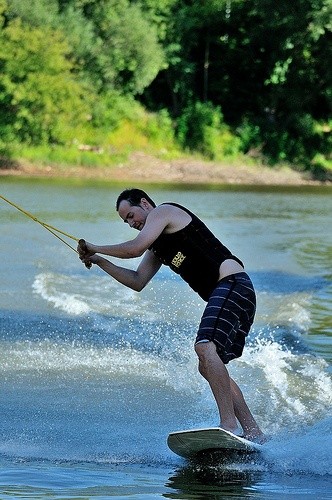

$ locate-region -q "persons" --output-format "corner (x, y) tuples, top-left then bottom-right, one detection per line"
(76, 188), (271, 449)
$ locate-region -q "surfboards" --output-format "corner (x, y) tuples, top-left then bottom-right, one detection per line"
(168, 427), (262, 459)
